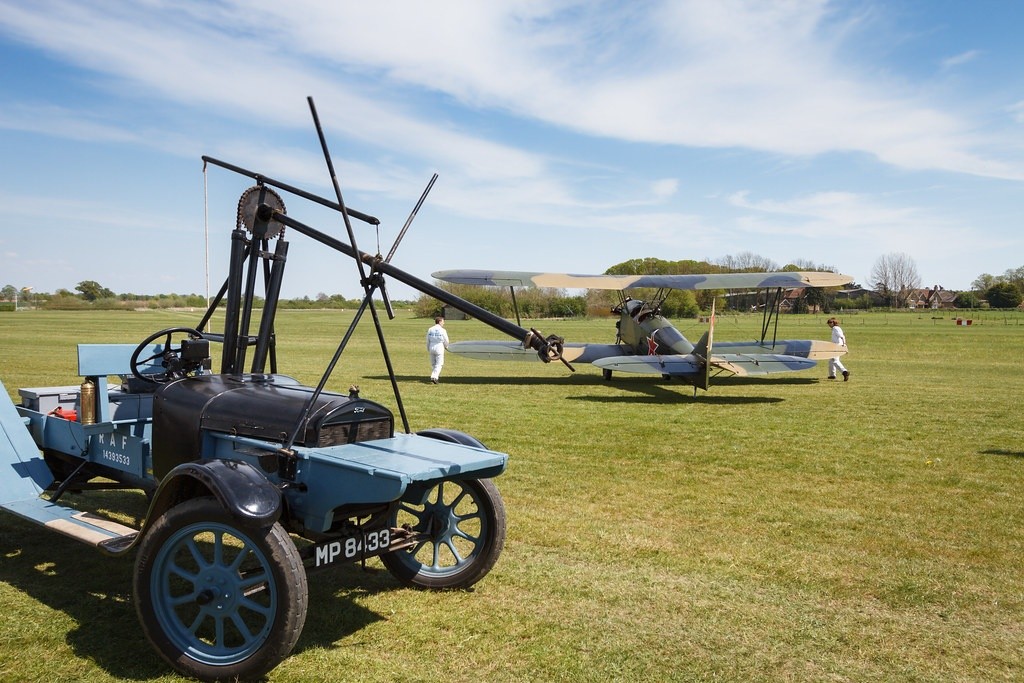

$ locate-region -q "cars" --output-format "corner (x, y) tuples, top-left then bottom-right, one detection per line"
(0, 95), (578, 682)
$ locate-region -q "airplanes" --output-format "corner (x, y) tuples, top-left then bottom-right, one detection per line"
(429, 270), (853, 392)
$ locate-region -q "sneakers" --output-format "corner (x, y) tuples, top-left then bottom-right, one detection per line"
(842, 370), (850, 380)
(430, 378), (440, 384)
(828, 376), (836, 379)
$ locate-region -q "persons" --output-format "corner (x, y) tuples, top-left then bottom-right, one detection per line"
(426, 317), (450, 385)
(826, 317), (850, 382)
